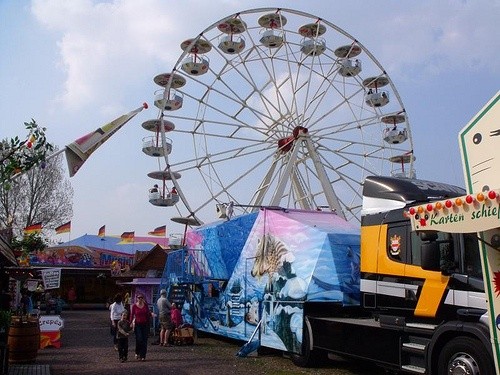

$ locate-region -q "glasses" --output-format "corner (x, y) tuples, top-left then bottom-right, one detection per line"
(137, 297), (144, 299)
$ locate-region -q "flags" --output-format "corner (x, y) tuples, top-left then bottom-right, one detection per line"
(148, 225), (166, 236)
(55, 221), (70, 234)
(98, 225), (105, 237)
(121, 232), (134, 243)
(23, 222), (42, 234)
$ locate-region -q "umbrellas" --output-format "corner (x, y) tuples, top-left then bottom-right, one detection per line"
(171, 217), (202, 246)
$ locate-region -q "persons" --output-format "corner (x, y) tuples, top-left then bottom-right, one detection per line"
(153, 303), (181, 345)
(367, 89), (373, 95)
(157, 289), (174, 347)
(355, 59), (360, 67)
(129, 294), (157, 361)
(171, 187), (177, 194)
(116, 310), (133, 363)
(0, 287), (77, 316)
(106, 292), (131, 351)
(151, 184), (158, 193)
(382, 92), (387, 98)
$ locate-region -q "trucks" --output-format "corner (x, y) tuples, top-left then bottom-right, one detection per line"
(286, 176), (496, 375)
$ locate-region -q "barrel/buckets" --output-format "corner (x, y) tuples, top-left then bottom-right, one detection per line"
(6, 315), (40, 361)
